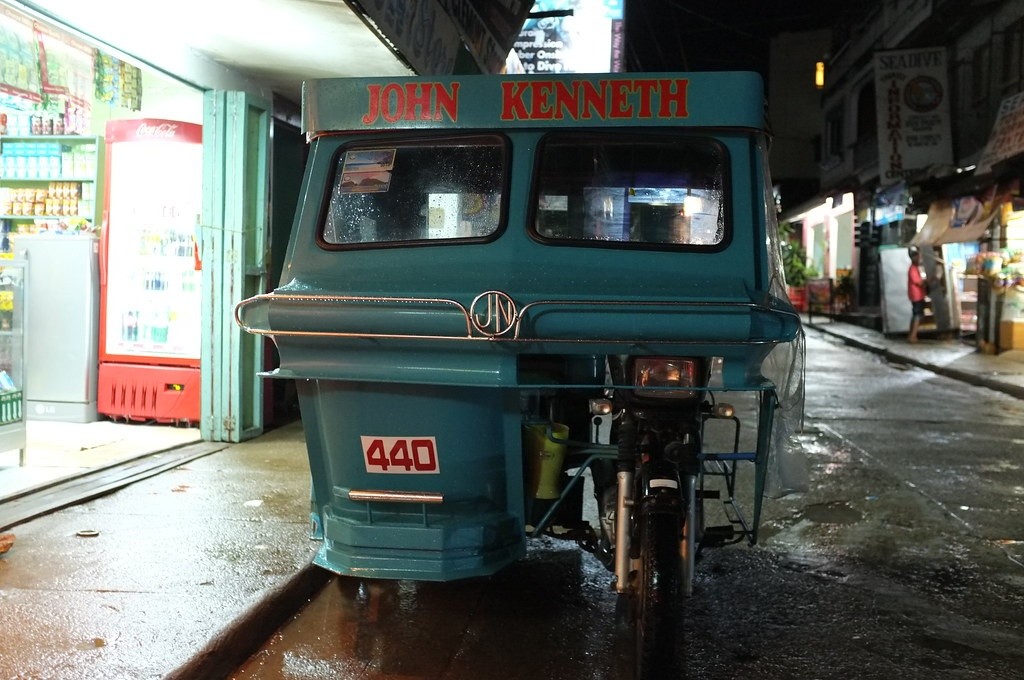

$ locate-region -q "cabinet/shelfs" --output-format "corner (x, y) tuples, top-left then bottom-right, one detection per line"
(0, 5), (141, 468)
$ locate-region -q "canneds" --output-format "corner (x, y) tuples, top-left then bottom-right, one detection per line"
(4, 181), (80, 217)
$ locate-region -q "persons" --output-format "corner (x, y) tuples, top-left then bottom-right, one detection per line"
(908, 246), (929, 344)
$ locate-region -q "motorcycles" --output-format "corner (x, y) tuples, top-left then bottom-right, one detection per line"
(232, 72), (807, 679)
